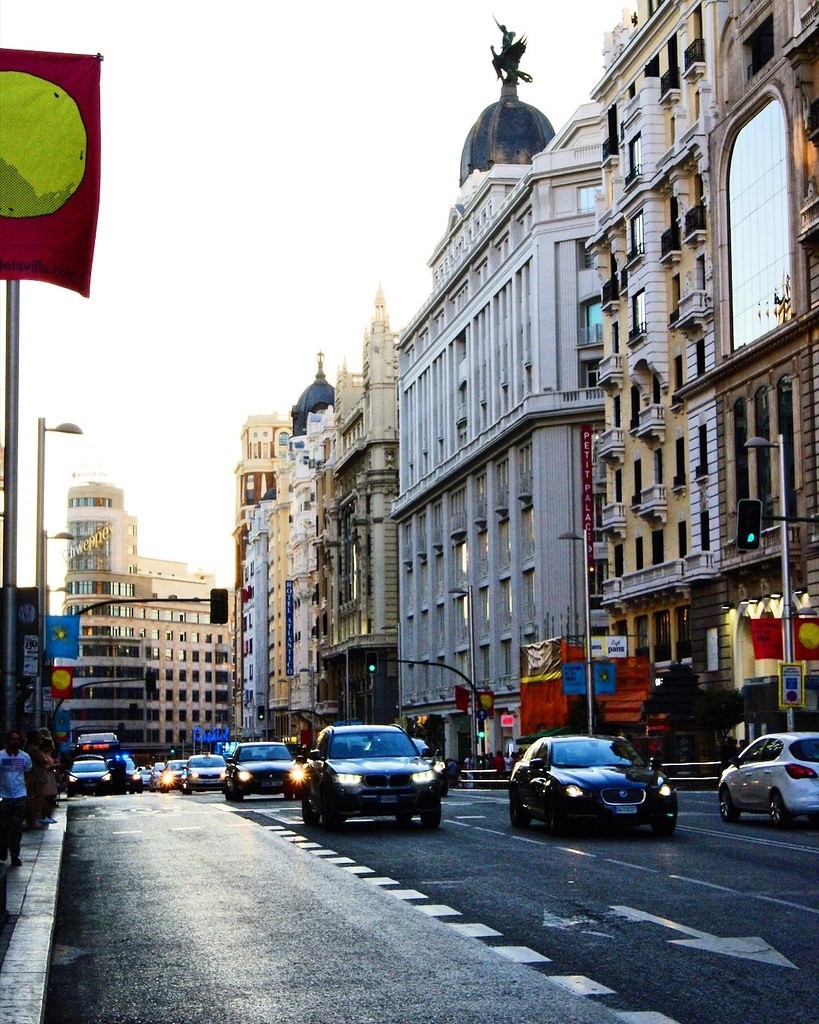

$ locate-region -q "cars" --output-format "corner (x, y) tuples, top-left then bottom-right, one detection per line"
(716, 731), (819, 828)
(123, 758), (143, 794)
(301, 724), (442, 829)
(506, 734), (679, 837)
(64, 759), (115, 797)
(140, 754), (227, 795)
(410, 738), (450, 796)
(223, 741), (303, 803)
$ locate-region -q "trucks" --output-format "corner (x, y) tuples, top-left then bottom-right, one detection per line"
(76, 732), (127, 795)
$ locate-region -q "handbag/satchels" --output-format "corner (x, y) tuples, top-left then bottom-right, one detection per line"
(40, 773), (58, 796)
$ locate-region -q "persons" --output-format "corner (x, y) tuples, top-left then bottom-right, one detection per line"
(723, 737), (746, 764)
(446, 748), (527, 789)
(20, 728), (63, 828)
(495, 20), (512, 52)
(0, 731), (31, 866)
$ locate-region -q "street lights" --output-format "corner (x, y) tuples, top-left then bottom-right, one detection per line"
(449, 585), (478, 789)
(36, 416), (84, 743)
(45, 583), (71, 617)
(743, 433), (795, 734)
(256, 665), (317, 749)
(557, 529), (594, 735)
(37, 530), (75, 737)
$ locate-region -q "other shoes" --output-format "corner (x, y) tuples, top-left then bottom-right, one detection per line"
(11, 855), (22, 866)
(27, 821), (44, 830)
(0, 842), (8, 862)
(39, 816), (57, 823)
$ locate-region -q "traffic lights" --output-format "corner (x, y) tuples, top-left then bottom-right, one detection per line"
(736, 499), (763, 551)
(170, 745), (176, 754)
(364, 651), (378, 674)
(333, 649), (350, 725)
(258, 706), (265, 721)
